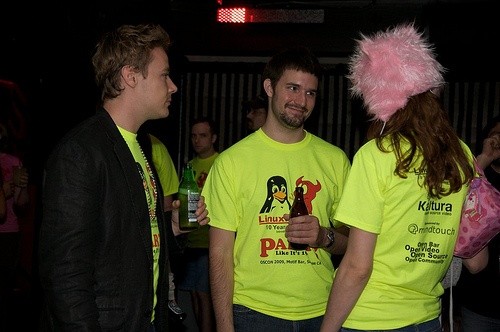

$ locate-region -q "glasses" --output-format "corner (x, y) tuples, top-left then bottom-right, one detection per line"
(246, 109), (268, 116)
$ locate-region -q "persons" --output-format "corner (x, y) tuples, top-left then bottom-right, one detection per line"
(180, 113), (220, 331)
(146, 130), (180, 212)
(200, 46), (352, 331)
(243, 91), (270, 132)
(0, 150), (35, 275)
(473, 124), (499, 186)
(42, 18), (211, 331)
(318, 21), (499, 331)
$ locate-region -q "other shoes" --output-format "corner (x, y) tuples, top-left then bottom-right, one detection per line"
(167, 301), (184, 314)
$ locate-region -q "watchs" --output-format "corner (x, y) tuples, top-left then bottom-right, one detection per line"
(324, 226), (337, 249)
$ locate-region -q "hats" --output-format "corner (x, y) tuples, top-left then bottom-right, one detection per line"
(241, 94), (268, 108)
(345, 21), (447, 123)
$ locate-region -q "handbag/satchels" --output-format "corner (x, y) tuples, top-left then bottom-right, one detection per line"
(453, 159), (500, 259)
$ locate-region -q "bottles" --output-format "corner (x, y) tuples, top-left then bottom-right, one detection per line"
(179, 162), (200, 231)
(289, 187), (310, 251)
